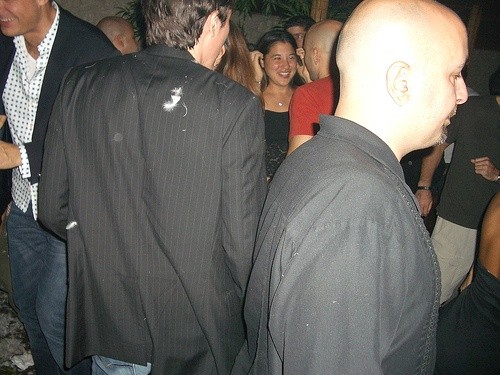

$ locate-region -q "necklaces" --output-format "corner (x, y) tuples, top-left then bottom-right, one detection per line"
(278, 101), (283, 106)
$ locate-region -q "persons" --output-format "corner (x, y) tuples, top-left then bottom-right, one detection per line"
(249, 30), (312, 182)
(39, 0), (267, 375)
(0, 0), (123, 375)
(434, 192), (500, 375)
(402, 145), (444, 234)
(284, 16), (317, 50)
(285, 20), (344, 156)
(211, 19), (265, 114)
(231, 0), (468, 375)
(415, 71), (500, 305)
(97, 16), (139, 55)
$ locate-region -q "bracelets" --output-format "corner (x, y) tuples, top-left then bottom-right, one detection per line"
(254, 80), (262, 84)
(495, 171), (500, 183)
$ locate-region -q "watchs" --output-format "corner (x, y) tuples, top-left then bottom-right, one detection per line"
(417, 186), (432, 190)
